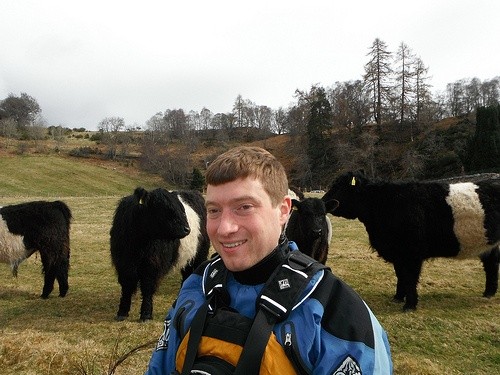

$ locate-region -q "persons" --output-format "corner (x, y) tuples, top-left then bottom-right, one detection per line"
(144, 145), (395, 375)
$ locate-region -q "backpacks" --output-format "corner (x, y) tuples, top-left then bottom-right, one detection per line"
(175, 251), (333, 375)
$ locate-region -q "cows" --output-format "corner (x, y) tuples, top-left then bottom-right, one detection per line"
(321, 170), (500, 313)
(108, 187), (211, 323)
(284, 185), (340, 265)
(0, 200), (74, 299)
